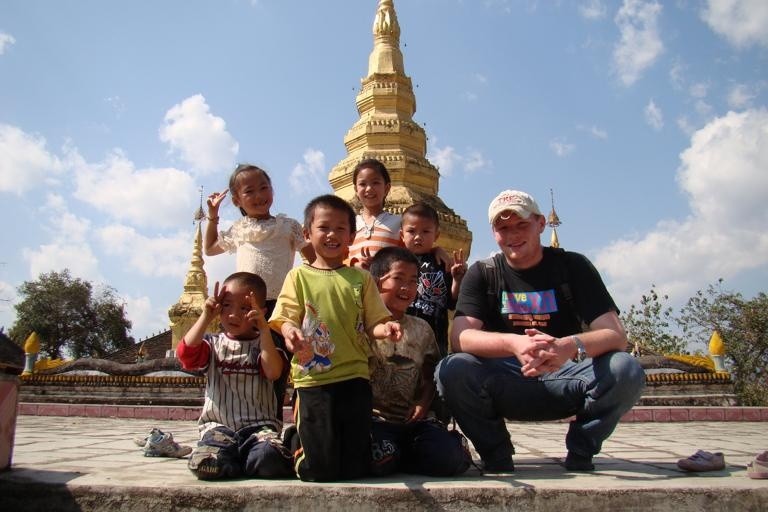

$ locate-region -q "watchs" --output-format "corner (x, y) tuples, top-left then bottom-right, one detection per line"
(571, 334), (588, 364)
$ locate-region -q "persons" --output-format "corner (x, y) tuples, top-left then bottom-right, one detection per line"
(174, 271), (294, 480)
(340, 157), (453, 277)
(201, 163), (318, 433)
(267, 195), (405, 483)
(370, 245), (473, 479)
(432, 187), (649, 476)
(361, 202), (469, 431)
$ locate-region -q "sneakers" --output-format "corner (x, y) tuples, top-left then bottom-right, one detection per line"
(746, 450), (768, 479)
(565, 450), (594, 471)
(133, 427), (192, 457)
(677, 449), (725, 472)
(480, 459), (514, 474)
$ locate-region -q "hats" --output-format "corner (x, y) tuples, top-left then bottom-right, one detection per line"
(488, 190), (542, 224)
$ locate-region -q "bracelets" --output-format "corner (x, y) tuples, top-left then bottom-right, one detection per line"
(207, 216), (219, 225)
